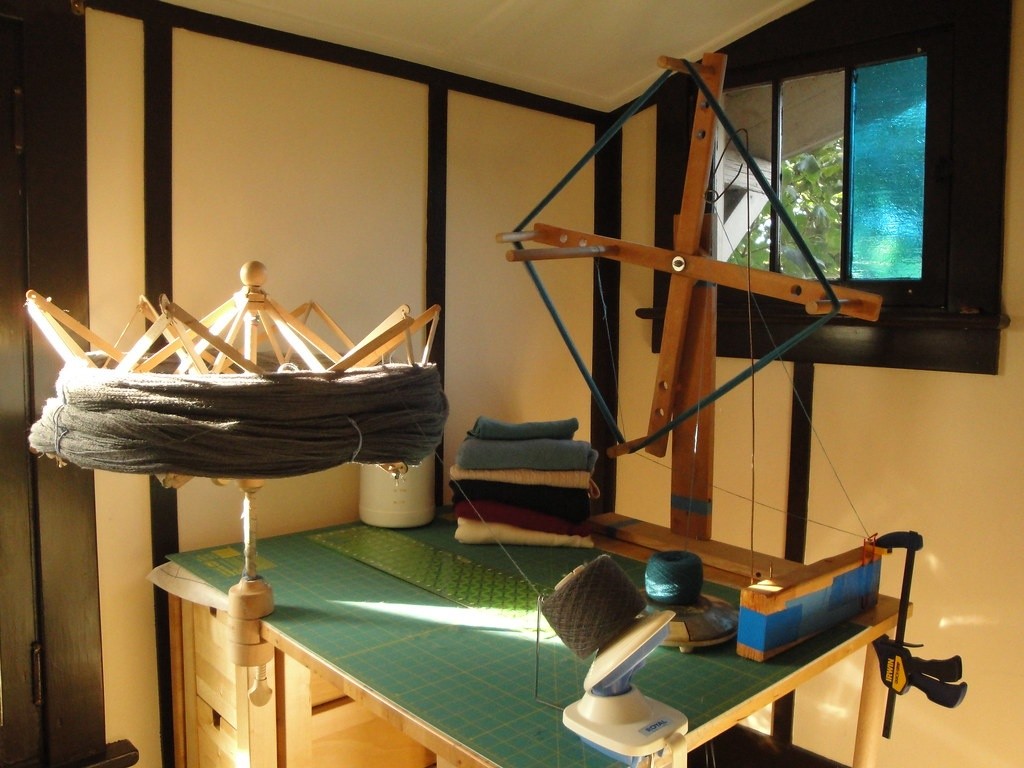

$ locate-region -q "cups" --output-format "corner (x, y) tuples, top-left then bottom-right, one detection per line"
(357, 454), (435, 528)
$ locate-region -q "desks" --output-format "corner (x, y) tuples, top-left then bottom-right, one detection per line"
(168, 510), (913, 768)
